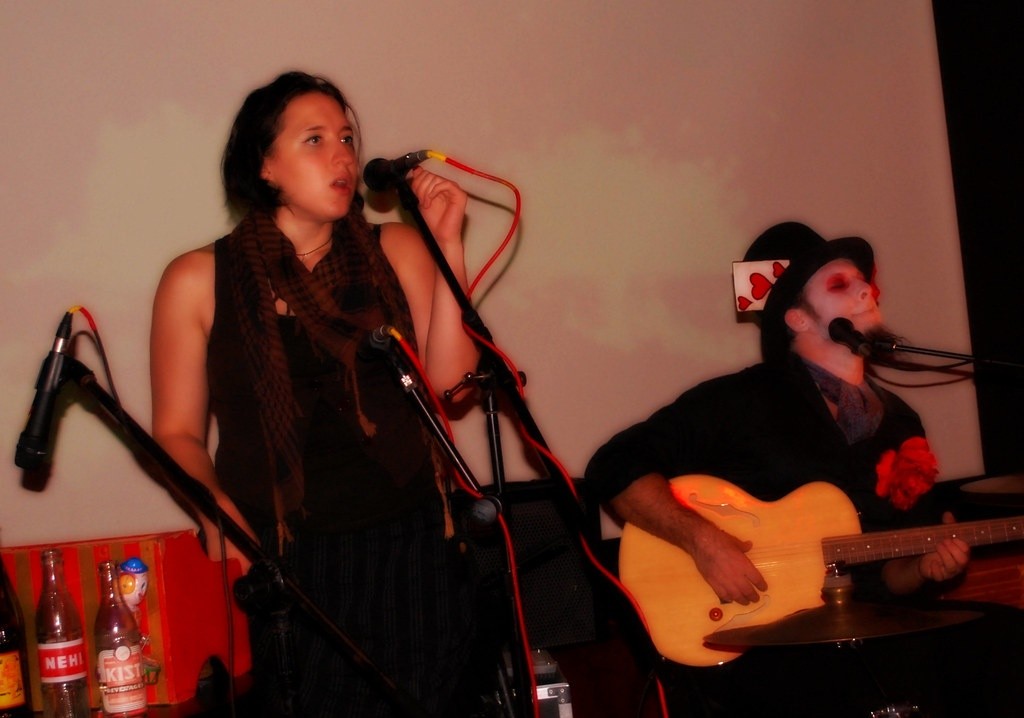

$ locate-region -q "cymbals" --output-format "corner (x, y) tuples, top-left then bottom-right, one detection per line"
(702, 601), (985, 647)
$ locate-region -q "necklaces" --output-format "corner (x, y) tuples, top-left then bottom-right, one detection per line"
(296, 238), (331, 256)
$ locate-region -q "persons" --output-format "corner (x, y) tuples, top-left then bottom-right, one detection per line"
(585, 221), (973, 716)
(149, 71), (477, 718)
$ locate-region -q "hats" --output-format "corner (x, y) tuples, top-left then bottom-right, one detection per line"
(732, 221), (874, 361)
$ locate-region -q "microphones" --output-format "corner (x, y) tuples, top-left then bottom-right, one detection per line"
(356, 326), (395, 364)
(14, 311), (72, 470)
(828, 318), (880, 364)
(362, 150), (433, 192)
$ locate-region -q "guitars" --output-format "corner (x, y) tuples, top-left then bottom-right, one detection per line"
(590, 467), (1022, 672)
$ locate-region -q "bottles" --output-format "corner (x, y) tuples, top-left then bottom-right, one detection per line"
(34, 547), (92, 718)
(95, 559), (148, 718)
(0, 556), (33, 718)
